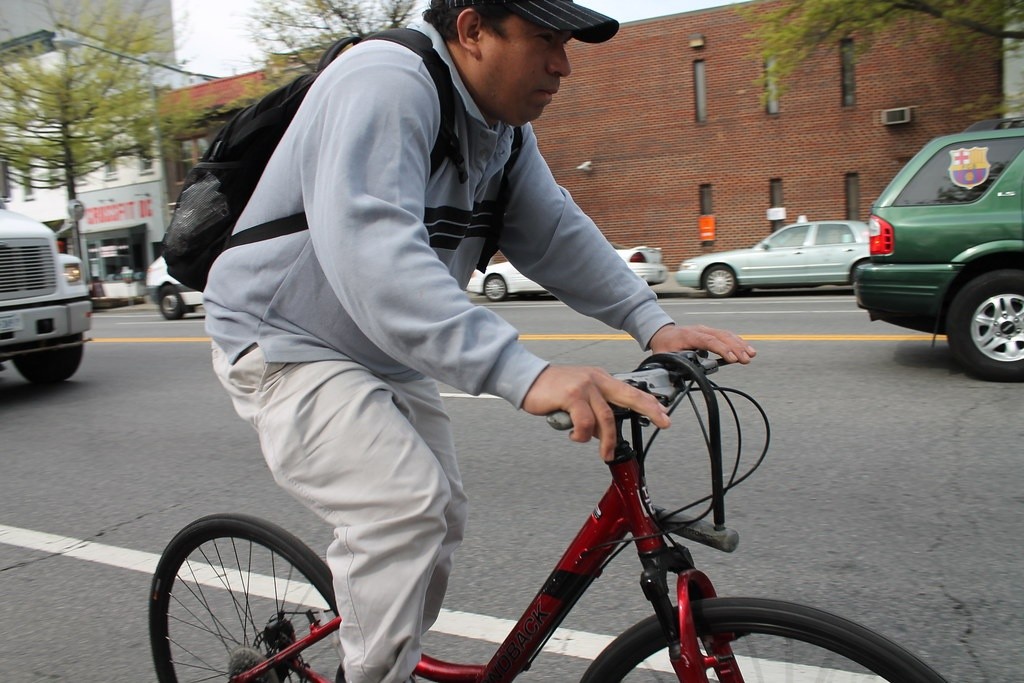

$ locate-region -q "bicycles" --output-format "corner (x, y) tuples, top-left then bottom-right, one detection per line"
(147, 346), (948, 683)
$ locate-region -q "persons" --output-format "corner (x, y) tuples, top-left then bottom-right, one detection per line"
(204, 0), (755, 683)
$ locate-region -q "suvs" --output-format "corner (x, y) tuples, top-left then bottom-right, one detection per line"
(850, 116), (1024, 384)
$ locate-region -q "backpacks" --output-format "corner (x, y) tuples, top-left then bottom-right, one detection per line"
(161, 27), (523, 292)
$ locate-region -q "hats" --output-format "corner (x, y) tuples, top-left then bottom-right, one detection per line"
(430, 0), (619, 44)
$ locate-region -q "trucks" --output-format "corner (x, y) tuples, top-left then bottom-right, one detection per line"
(0, 151), (95, 386)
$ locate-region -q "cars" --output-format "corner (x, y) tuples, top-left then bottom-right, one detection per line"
(145, 255), (203, 322)
(675, 213), (870, 299)
(465, 238), (669, 303)
